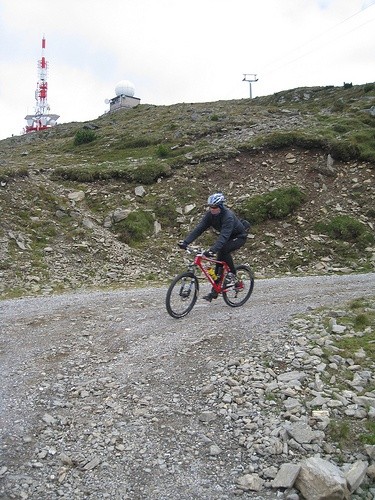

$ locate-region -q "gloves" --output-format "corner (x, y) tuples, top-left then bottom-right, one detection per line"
(180, 241), (187, 249)
(205, 250), (214, 257)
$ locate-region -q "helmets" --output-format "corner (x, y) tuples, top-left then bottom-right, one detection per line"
(207, 193), (225, 205)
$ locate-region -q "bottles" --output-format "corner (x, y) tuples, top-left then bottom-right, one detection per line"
(207, 267), (218, 281)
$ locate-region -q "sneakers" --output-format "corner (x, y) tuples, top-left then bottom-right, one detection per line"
(223, 273), (239, 290)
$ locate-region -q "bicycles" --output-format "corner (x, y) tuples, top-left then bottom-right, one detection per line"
(165, 240), (254, 319)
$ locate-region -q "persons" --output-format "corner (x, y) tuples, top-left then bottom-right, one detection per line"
(178, 193), (247, 302)
